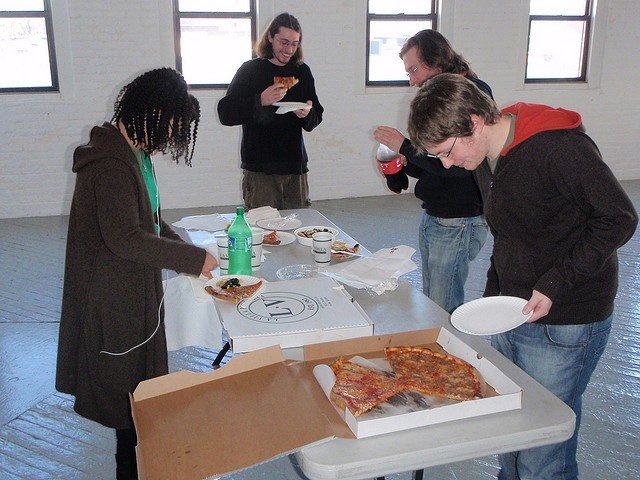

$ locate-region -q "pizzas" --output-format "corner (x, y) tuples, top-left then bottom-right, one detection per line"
(263, 229), (282, 244)
(205, 281), (263, 302)
(274, 77), (299, 91)
(384, 347), (487, 402)
(330, 345), (400, 417)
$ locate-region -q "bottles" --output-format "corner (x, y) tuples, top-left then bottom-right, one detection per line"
(376, 143), (409, 194)
(228, 207), (252, 275)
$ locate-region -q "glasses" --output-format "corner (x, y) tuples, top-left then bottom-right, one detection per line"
(272, 32), (301, 49)
(406, 60), (426, 76)
(426, 130), (459, 160)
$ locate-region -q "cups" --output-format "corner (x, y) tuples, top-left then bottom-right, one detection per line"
(251, 227), (263, 271)
(312, 232), (333, 266)
(216, 239), (229, 275)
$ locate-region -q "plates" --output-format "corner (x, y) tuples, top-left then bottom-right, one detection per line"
(259, 231), (296, 246)
(347, 244), (362, 259)
(276, 264), (327, 280)
(450, 296), (534, 336)
(272, 102), (309, 107)
(257, 218), (302, 230)
(204, 275), (265, 303)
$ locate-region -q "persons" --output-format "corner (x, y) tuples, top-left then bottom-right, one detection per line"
(407, 73), (638, 480)
(218, 13), (325, 213)
(55, 68), (219, 479)
(374, 29), (489, 314)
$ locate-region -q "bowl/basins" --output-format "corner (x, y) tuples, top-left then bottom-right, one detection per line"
(294, 226), (339, 246)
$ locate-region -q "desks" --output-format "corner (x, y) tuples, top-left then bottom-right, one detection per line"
(180, 208), (577, 476)
